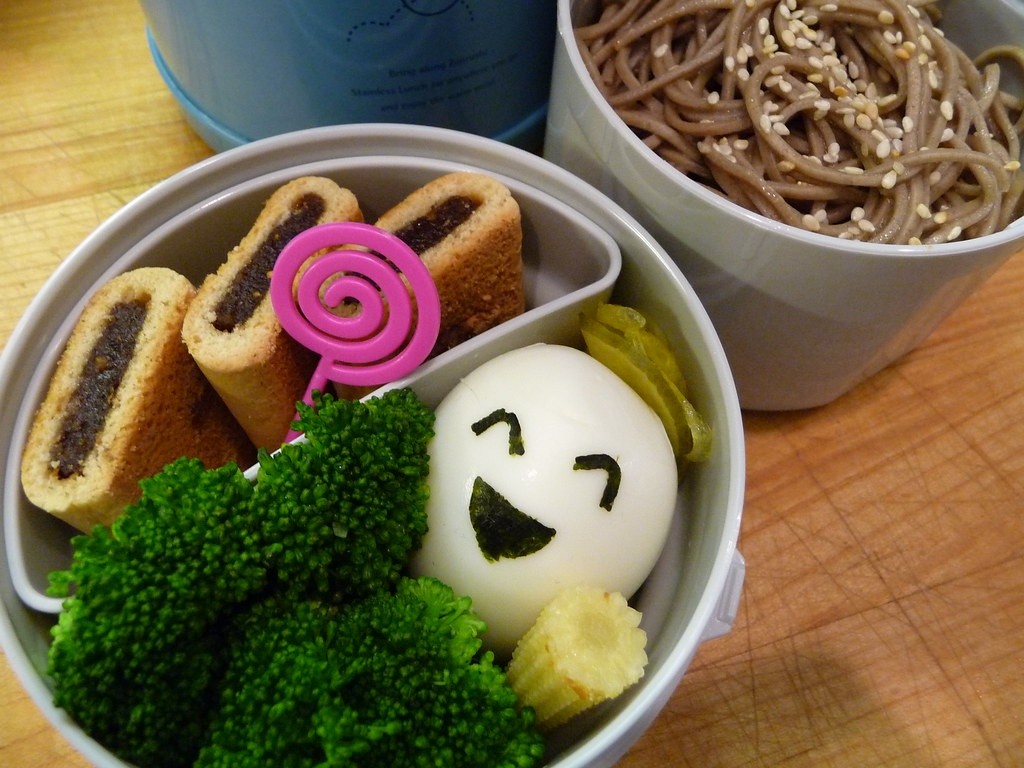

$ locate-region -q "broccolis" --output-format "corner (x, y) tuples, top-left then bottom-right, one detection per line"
(42, 381), (546, 768)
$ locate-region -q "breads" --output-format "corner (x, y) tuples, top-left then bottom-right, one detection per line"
(181, 176), (366, 454)
(20, 268), (255, 531)
(323, 172), (526, 404)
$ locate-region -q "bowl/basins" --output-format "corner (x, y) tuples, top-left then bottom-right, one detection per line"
(0, 123), (748, 768)
(540, 0), (1024, 413)
(134, 0), (557, 175)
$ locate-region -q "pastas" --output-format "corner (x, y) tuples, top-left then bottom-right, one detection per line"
(573, 0), (1024, 245)
